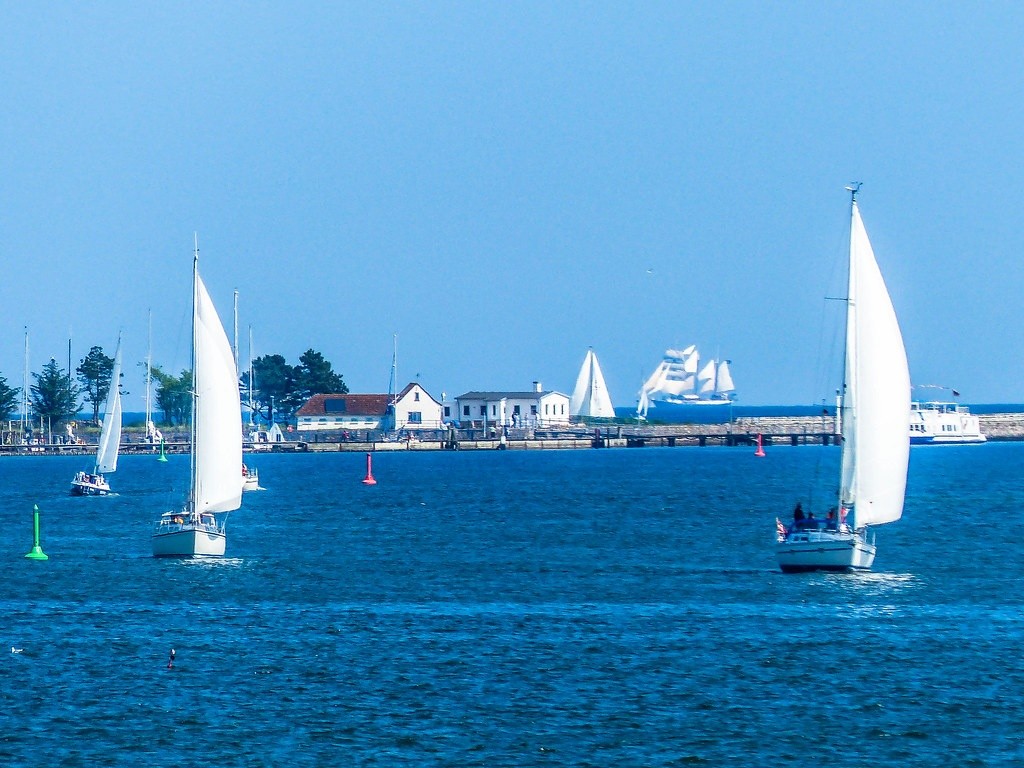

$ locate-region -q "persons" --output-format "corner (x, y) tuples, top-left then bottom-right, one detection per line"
(795, 501), (838, 531)
(342, 429), (350, 442)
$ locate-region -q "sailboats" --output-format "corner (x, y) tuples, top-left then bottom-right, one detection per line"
(568, 344), (620, 426)
(639, 346), (739, 422)
(71, 330), (123, 496)
(150, 229), (246, 558)
(772, 180), (914, 572)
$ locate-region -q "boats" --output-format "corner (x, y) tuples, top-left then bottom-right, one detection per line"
(240, 465), (260, 491)
(909, 400), (988, 445)
